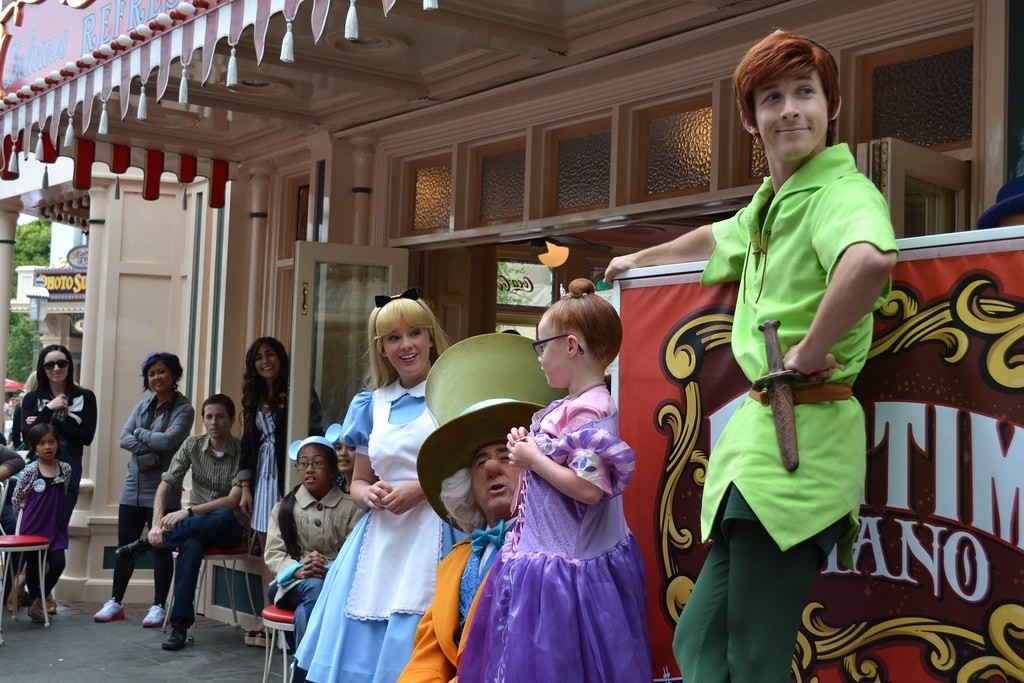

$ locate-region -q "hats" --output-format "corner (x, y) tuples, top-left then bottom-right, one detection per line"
(978, 176), (1024, 228)
(416, 333), (570, 533)
(288, 424), (342, 460)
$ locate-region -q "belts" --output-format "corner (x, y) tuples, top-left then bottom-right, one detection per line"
(750, 383), (853, 407)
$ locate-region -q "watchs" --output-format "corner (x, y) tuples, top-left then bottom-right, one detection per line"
(183, 505), (194, 517)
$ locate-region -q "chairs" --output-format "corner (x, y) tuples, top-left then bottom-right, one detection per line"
(160, 516), (259, 640)
(0, 481), (50, 631)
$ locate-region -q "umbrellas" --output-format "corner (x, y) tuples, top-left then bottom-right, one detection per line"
(4, 377), (27, 393)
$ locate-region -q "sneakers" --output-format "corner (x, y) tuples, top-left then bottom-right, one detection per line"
(115, 537), (147, 564)
(143, 603), (167, 627)
(162, 629), (187, 650)
(94, 597), (125, 621)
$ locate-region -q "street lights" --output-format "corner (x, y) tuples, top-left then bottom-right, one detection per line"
(24, 285), (52, 371)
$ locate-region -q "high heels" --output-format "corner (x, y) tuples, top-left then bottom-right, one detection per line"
(255, 632), (278, 647)
(244, 629), (264, 645)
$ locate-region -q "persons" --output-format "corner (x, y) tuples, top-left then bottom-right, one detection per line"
(292, 286), (470, 683)
(456, 278), (655, 683)
(0, 433), (26, 482)
(94, 351), (196, 630)
(393, 440), (526, 683)
(3, 344), (98, 614)
(11, 423), (72, 623)
(236, 333), (323, 649)
(603, 27), (898, 683)
(116, 394), (255, 651)
(973, 174), (1024, 230)
(264, 408), (369, 651)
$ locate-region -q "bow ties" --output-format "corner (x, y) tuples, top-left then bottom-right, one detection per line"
(470, 519), (506, 557)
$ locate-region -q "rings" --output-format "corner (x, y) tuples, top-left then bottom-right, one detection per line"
(63, 404), (65, 407)
(155, 538), (157, 540)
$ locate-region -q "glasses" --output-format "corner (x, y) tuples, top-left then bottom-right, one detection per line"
(532, 335), (584, 357)
(294, 460), (332, 469)
(43, 359), (69, 370)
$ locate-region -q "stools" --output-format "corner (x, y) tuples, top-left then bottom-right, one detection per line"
(262, 604), (296, 683)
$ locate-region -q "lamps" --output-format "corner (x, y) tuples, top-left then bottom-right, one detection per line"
(535, 237), (570, 268)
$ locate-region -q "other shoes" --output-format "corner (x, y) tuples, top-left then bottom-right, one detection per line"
(27, 610), (50, 622)
(6, 574), (24, 612)
(46, 593), (56, 614)
